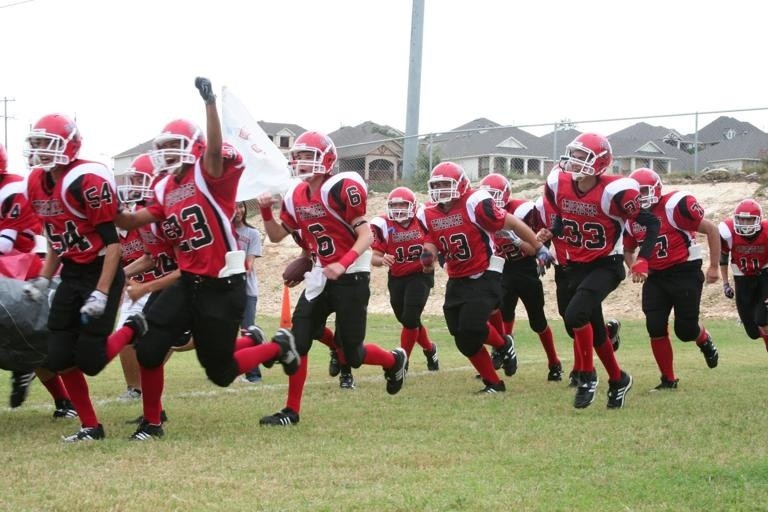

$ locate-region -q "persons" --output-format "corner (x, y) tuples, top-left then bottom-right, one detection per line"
(622, 168), (722, 392)
(717, 199), (768, 350)
(371, 132), (660, 411)
(258, 129), (408, 427)
(0, 77), (301, 441)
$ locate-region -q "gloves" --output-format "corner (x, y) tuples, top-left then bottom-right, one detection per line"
(723, 282), (735, 299)
(78, 288), (110, 324)
(536, 245), (556, 276)
(21, 274), (51, 306)
(194, 76), (216, 105)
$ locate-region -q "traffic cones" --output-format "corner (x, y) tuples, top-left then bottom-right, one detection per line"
(280, 282), (292, 329)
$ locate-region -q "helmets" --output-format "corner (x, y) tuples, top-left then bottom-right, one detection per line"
(24, 114), (81, 171)
(287, 130), (337, 180)
(732, 198), (763, 237)
(151, 117), (206, 172)
(385, 186), (418, 222)
(558, 132), (613, 178)
(477, 172), (513, 208)
(425, 161), (472, 205)
(117, 152), (156, 204)
(625, 166), (663, 211)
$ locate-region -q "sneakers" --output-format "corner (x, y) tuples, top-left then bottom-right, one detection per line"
(259, 406), (300, 426)
(491, 334), (517, 377)
(240, 324), (301, 376)
(62, 424), (106, 441)
(647, 374), (680, 394)
(472, 379), (506, 397)
(548, 362), (562, 381)
(605, 319), (621, 353)
(567, 369), (599, 410)
(382, 346), (407, 396)
(124, 313), (147, 347)
(605, 369), (633, 410)
(421, 342), (439, 371)
(328, 348), (354, 389)
(10, 367), (37, 408)
(696, 331), (719, 369)
(115, 386), (143, 404)
(52, 399), (79, 422)
(125, 408), (169, 442)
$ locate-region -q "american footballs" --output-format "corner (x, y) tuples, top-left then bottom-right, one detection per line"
(283, 257), (312, 284)
